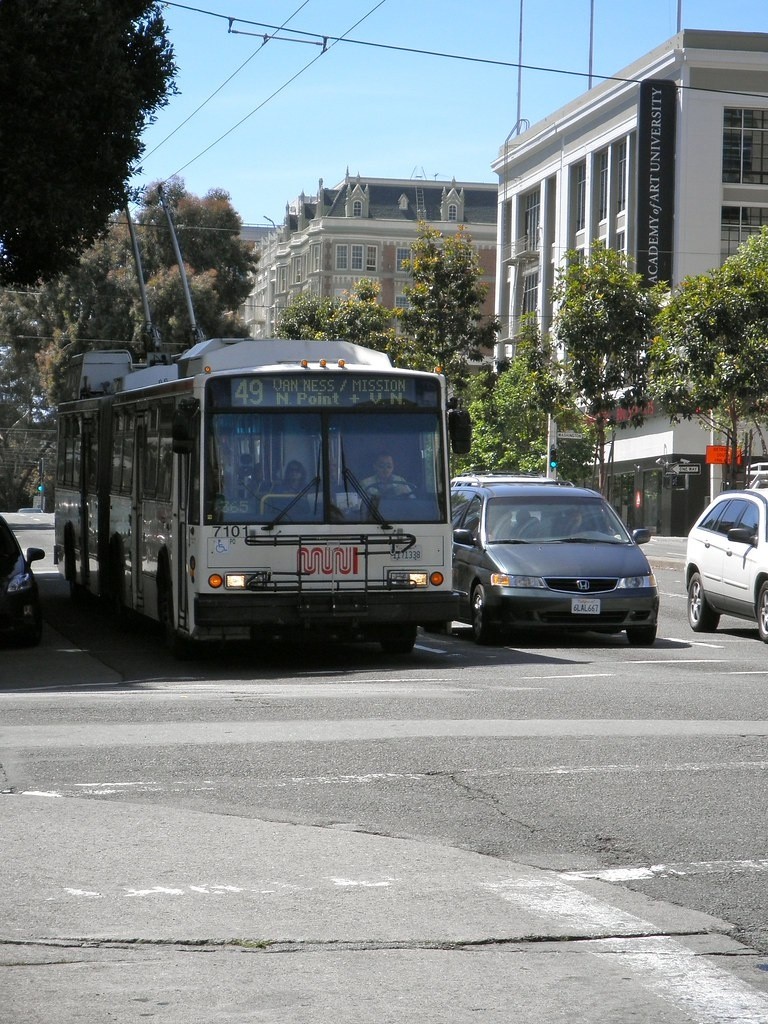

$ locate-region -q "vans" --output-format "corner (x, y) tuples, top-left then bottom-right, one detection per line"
(424, 486), (661, 644)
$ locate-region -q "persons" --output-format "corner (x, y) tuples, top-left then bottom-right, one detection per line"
(277, 461), (311, 515)
(356, 451), (418, 498)
(560, 511), (590, 537)
(514, 511), (544, 539)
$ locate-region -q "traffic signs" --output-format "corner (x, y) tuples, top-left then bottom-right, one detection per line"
(671, 464), (701, 475)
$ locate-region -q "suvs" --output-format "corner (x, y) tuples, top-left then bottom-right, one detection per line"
(451, 473), (576, 520)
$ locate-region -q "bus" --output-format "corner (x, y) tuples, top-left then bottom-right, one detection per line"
(50, 337), (470, 653)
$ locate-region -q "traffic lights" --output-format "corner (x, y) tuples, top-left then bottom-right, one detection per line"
(37, 479), (42, 491)
(548, 449), (557, 469)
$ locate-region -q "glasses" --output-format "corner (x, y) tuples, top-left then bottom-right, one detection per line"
(376, 462), (392, 470)
(287, 468), (303, 474)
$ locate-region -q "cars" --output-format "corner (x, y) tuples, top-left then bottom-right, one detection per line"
(685, 487), (768, 642)
(18, 508), (43, 513)
(0, 514), (46, 653)
